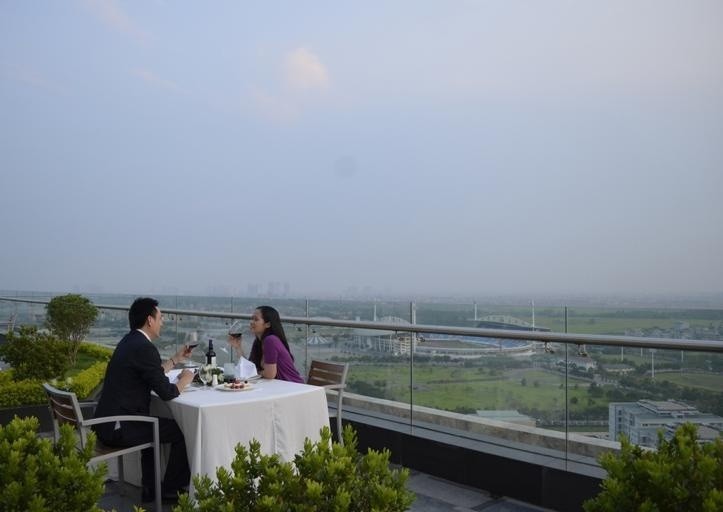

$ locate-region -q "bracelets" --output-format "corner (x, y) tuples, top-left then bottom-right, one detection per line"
(169, 358), (175, 366)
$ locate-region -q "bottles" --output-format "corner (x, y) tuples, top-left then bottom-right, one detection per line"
(204, 339), (217, 366)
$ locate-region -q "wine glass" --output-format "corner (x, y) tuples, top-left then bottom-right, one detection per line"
(222, 322), (242, 351)
(184, 328), (197, 367)
(199, 366), (211, 391)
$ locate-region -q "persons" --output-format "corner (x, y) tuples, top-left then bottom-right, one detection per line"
(225, 305), (305, 384)
(88, 296), (192, 504)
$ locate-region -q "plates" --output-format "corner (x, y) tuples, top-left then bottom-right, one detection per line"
(216, 384), (256, 391)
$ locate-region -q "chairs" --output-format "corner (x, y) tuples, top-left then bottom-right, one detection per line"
(42, 383), (162, 512)
(307, 359), (350, 447)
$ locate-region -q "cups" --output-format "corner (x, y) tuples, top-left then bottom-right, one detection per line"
(223, 363), (235, 383)
